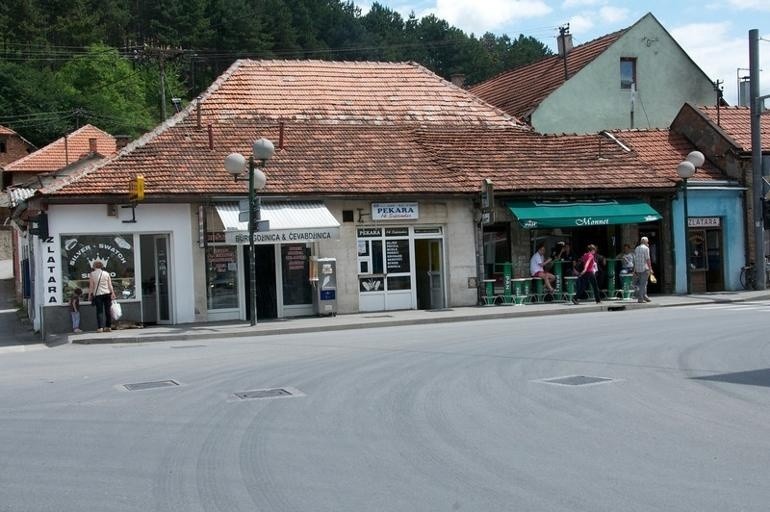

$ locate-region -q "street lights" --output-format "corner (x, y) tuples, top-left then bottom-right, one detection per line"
(225, 138), (275, 326)
(677, 151), (705, 294)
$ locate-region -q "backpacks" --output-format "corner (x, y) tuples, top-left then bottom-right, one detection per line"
(575, 259), (585, 273)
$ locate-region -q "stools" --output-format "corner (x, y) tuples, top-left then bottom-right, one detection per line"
(482, 273), (635, 307)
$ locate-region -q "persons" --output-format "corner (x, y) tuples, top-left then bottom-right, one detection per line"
(570, 244), (602, 305)
(529, 246), (559, 295)
(634, 236), (654, 302)
(558, 243), (634, 283)
(68, 288), (84, 333)
(87, 261), (116, 332)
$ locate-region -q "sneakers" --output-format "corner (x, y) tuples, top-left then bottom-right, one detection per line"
(594, 300), (604, 304)
(96, 328), (111, 332)
(637, 296), (653, 303)
(572, 298), (580, 305)
(74, 328), (83, 333)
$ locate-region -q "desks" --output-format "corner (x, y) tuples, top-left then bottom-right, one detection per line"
(495, 259), (624, 306)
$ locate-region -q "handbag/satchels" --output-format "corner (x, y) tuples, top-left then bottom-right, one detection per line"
(109, 300), (123, 321)
(92, 297), (96, 305)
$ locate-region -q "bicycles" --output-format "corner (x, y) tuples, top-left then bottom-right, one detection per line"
(740, 255), (770, 290)
